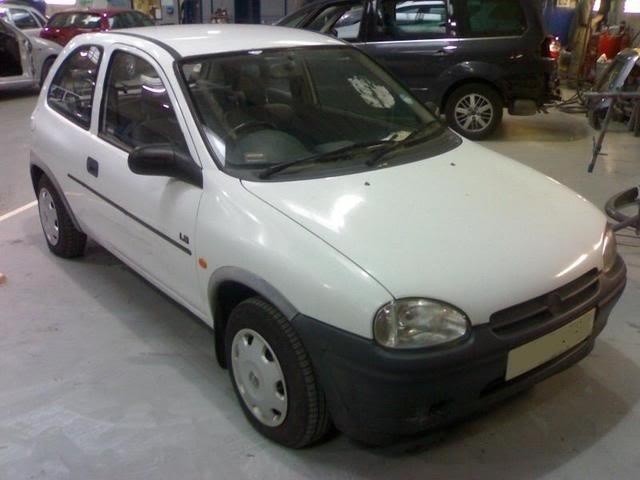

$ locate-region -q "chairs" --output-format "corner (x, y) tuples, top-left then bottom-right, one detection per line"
(134, 59), (302, 153)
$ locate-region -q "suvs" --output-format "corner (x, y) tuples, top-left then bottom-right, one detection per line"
(139, 0), (564, 140)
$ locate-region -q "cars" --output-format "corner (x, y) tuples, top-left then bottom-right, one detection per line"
(30, 0), (626, 452)
(39, 7), (160, 80)
(0, 3), (63, 93)
